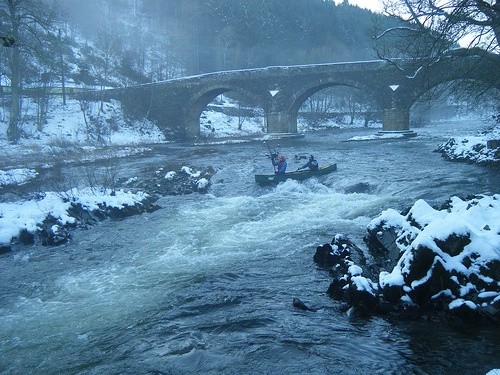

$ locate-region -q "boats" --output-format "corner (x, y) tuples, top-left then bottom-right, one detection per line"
(254, 162), (337, 187)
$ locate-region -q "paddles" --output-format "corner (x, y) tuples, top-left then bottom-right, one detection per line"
(265, 140), (276, 172)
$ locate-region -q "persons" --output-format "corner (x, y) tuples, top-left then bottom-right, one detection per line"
(270, 155), (287, 174)
(298, 155), (318, 170)
